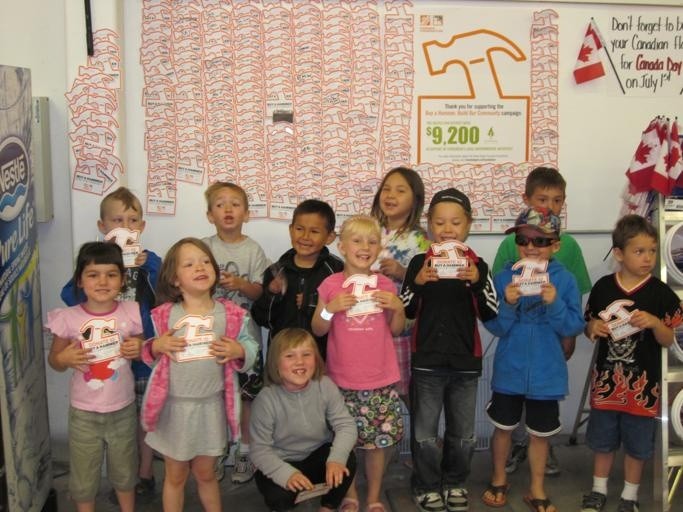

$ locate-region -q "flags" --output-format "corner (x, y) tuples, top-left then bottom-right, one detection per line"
(573, 19), (607, 84)
(624, 115), (683, 210)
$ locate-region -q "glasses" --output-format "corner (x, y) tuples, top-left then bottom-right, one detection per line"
(514, 234), (552, 247)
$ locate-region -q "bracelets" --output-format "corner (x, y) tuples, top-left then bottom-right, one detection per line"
(318, 306), (335, 321)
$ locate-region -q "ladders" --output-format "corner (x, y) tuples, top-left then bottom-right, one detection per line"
(568, 132), (681, 512)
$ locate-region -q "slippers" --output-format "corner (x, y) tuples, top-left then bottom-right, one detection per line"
(482, 481), (511, 506)
(524, 495), (552, 511)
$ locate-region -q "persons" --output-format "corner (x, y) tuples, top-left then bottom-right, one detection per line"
(492, 165), (591, 477)
(581, 214), (683, 512)
(60, 187), (163, 495)
(311, 213), (406, 511)
(43, 240), (144, 512)
(140, 236), (258, 511)
(251, 199), (344, 364)
(247, 328), (356, 510)
(198, 181), (268, 485)
(482, 206), (586, 511)
(370, 167), (441, 447)
(399, 185), (500, 512)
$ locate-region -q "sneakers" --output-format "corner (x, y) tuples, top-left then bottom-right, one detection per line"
(444, 489), (468, 511)
(232, 449), (255, 484)
(111, 477), (154, 504)
(582, 491), (606, 512)
(618, 496), (638, 512)
(544, 448), (560, 475)
(215, 446), (229, 481)
(414, 491), (446, 512)
(506, 446), (525, 474)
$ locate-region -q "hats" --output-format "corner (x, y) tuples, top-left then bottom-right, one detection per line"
(505, 206), (559, 237)
(430, 189), (471, 212)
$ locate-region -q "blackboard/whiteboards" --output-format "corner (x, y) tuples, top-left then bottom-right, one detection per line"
(409, 0), (683, 236)
